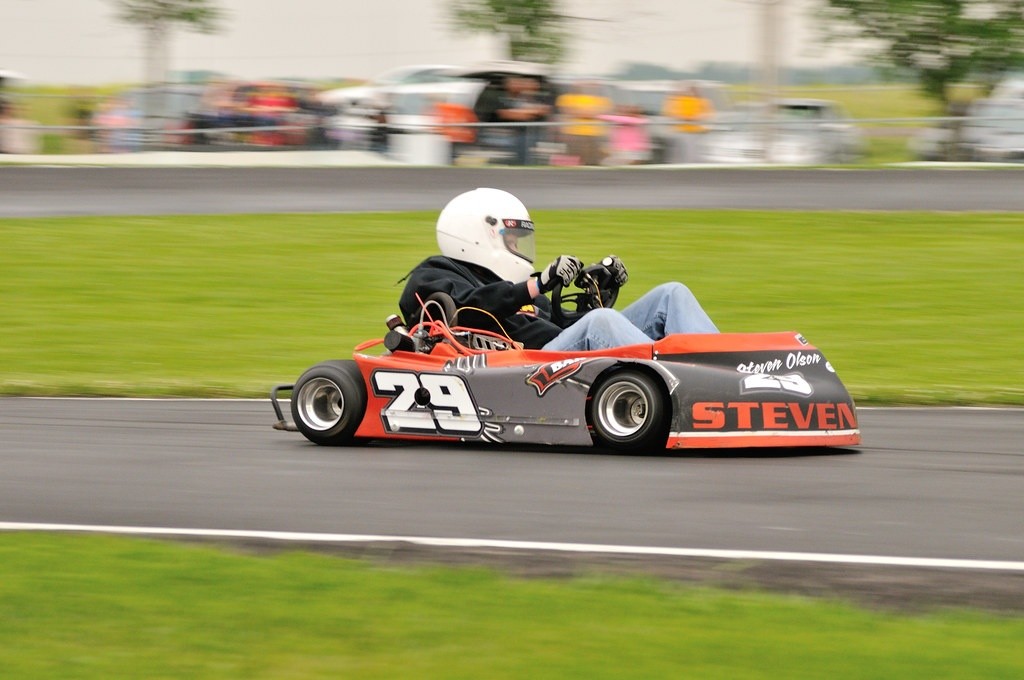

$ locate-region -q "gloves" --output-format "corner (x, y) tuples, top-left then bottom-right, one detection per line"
(540, 255), (584, 294)
(592, 255), (629, 289)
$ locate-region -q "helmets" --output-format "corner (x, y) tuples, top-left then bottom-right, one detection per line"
(436, 187), (536, 285)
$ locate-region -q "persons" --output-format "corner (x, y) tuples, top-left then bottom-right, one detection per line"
(400, 187), (721, 351)
(473, 75), (557, 166)
